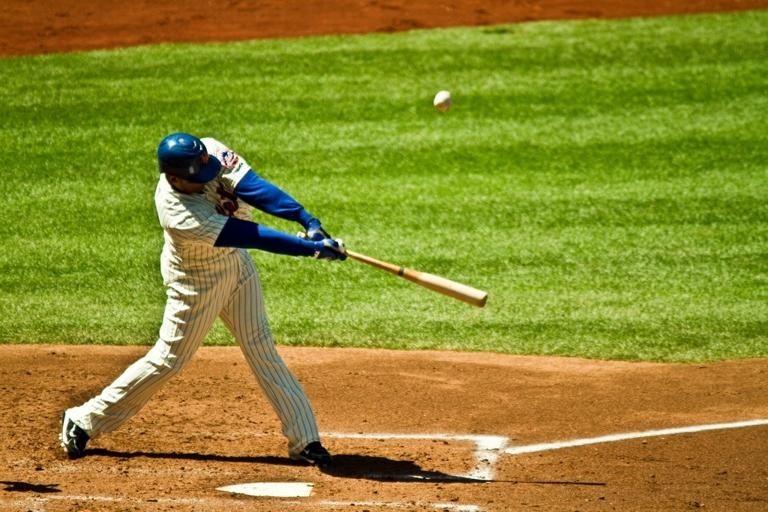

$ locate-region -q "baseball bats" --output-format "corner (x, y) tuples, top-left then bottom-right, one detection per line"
(297, 231), (487, 307)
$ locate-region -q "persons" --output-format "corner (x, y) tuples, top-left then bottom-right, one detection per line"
(58, 133), (347, 466)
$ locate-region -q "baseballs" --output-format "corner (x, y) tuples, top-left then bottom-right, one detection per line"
(432, 90), (450, 112)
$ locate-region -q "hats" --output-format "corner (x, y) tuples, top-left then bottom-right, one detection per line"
(159, 133), (221, 183)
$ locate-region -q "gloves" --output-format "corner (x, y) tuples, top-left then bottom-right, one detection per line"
(306, 222), (331, 240)
(314, 239), (346, 261)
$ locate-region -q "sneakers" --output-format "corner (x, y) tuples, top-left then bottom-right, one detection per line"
(290, 442), (330, 463)
(59, 411), (89, 457)
